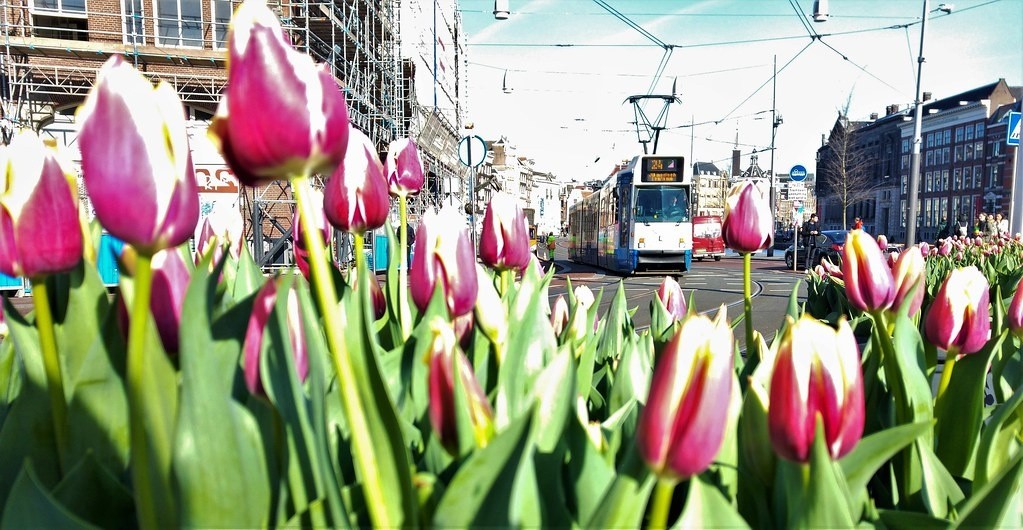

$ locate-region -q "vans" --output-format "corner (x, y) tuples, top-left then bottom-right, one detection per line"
(693, 215), (725, 261)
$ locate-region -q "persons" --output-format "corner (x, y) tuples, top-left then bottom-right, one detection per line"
(782, 222), (801, 241)
(397, 218), (415, 274)
(973, 212), (1008, 236)
(854, 216), (861, 223)
(935, 217), (947, 242)
(800, 213), (820, 274)
(955, 214), (968, 236)
(669, 196), (677, 207)
(547, 232), (556, 262)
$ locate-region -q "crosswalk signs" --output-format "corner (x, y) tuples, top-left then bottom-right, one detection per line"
(1005, 112), (1021, 147)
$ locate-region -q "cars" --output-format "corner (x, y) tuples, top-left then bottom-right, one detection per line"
(783, 230), (850, 271)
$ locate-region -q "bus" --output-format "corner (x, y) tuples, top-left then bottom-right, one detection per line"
(529, 224), (537, 256)
(568, 94), (693, 282)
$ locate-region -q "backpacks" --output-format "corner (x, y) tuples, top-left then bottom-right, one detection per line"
(958, 221), (968, 237)
